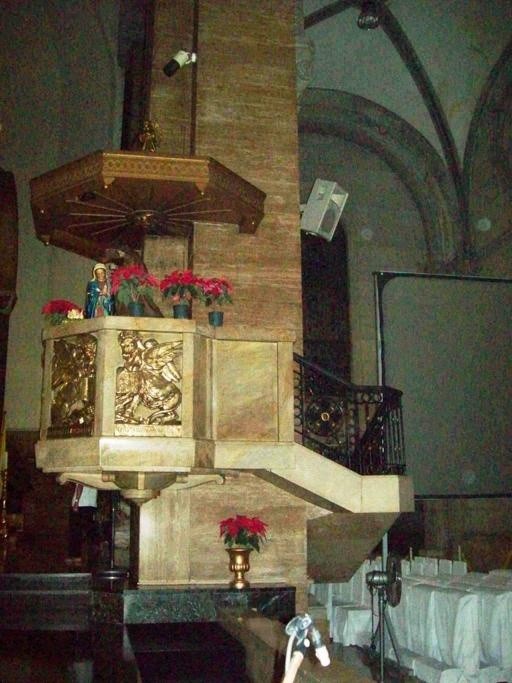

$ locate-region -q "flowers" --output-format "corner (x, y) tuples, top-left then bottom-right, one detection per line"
(199, 278), (233, 311)
(220, 515), (268, 553)
(110, 263), (161, 302)
(160, 270), (202, 307)
(42, 300), (81, 320)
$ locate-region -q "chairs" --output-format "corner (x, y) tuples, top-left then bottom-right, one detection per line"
(307, 556), (467, 647)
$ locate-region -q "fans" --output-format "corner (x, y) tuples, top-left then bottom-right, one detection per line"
(365, 554), (402, 682)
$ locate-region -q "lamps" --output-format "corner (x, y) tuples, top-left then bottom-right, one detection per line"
(357, 0), (380, 29)
(163, 49), (197, 77)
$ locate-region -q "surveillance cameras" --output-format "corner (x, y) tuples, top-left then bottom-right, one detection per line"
(163, 47), (198, 77)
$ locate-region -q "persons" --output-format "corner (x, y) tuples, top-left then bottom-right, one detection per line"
(85, 263), (114, 319)
(139, 120), (157, 153)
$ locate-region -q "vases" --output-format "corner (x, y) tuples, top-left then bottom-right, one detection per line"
(51, 319), (62, 326)
(225, 548), (254, 590)
(128, 302), (144, 317)
(173, 304), (192, 320)
(209, 312), (224, 327)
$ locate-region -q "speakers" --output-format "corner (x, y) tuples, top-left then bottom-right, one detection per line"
(300, 177), (348, 242)
